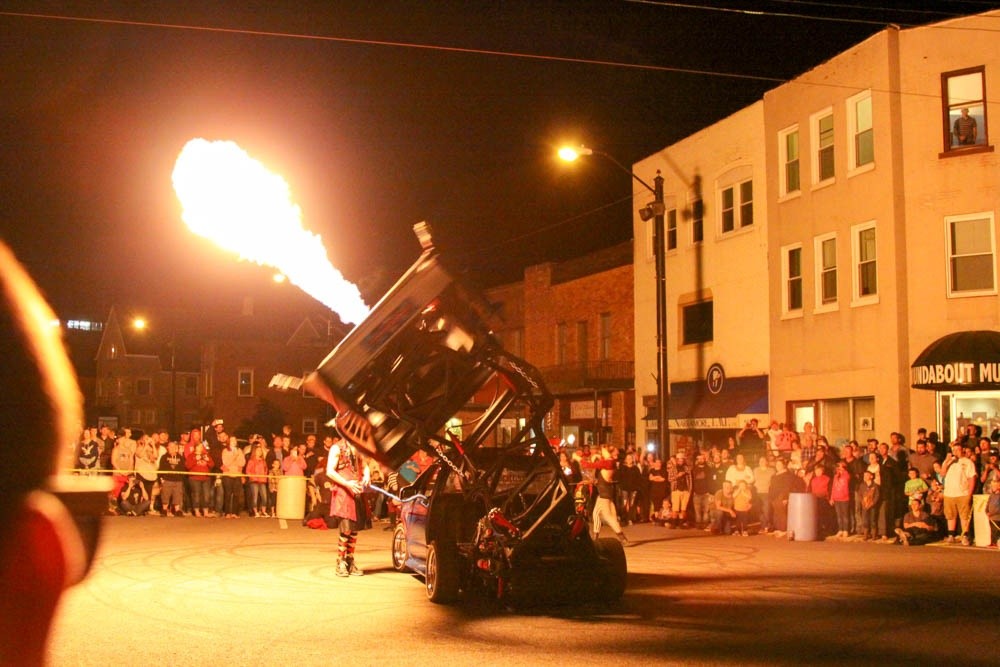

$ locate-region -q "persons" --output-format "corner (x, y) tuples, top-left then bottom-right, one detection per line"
(365, 450), (434, 531)
(72, 420), (338, 520)
(552, 419), (1000, 548)
(326, 439), (370, 576)
(954, 108), (978, 146)
(0, 273), (86, 667)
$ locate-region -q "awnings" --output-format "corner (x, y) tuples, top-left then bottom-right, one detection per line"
(641, 375), (769, 429)
(911, 330), (1000, 392)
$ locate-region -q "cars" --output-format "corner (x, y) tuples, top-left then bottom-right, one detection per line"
(391, 445), (628, 607)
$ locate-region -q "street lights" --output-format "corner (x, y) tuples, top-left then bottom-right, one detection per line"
(133, 318), (177, 441)
(560, 142), (679, 470)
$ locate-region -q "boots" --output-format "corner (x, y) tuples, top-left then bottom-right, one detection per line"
(618, 533), (628, 545)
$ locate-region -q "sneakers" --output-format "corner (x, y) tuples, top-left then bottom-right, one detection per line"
(335, 558), (349, 576)
(962, 535), (971, 546)
(347, 559), (363, 576)
(946, 536), (956, 543)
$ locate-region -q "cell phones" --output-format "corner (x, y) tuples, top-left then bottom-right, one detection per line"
(747, 424), (752, 427)
(293, 451), (297, 457)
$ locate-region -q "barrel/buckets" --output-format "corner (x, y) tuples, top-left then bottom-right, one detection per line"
(787, 493), (820, 540)
(277, 477), (307, 519)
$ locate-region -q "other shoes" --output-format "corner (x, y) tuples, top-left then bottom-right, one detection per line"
(107, 508), (277, 518)
(620, 516), (887, 543)
